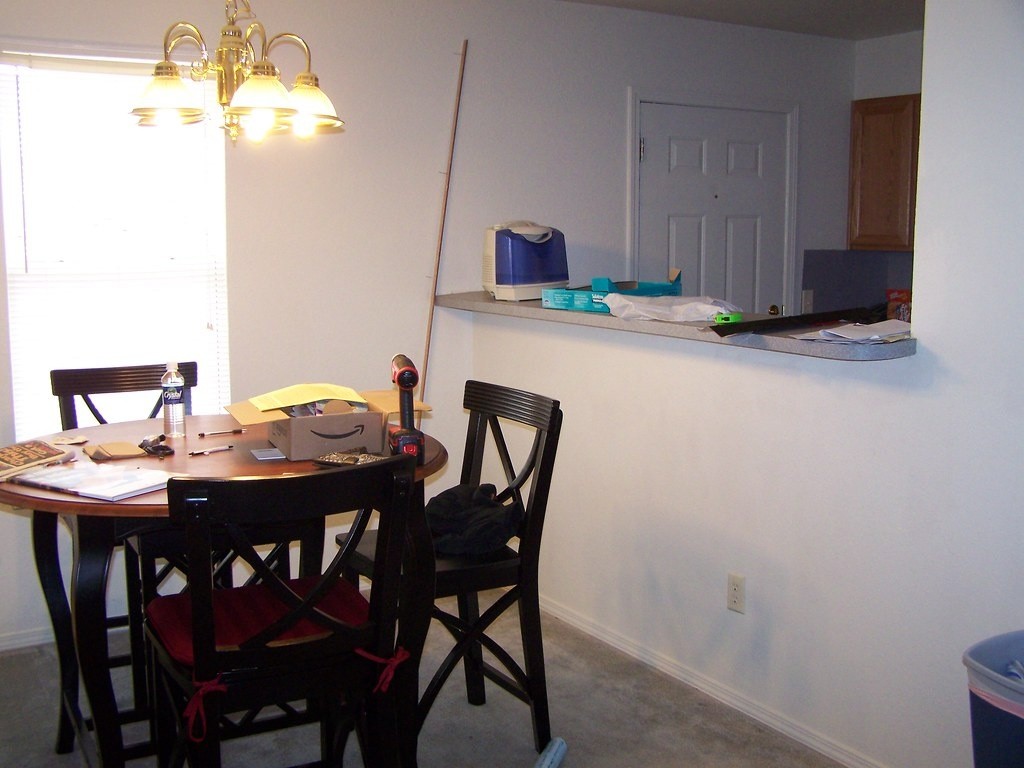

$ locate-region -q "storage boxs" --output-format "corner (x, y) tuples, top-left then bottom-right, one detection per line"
(885, 287), (913, 321)
(222, 380), (432, 460)
(542, 273), (682, 314)
(962, 628), (1024, 768)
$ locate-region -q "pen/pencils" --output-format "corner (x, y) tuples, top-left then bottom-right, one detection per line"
(198, 428), (247, 437)
(810, 320), (839, 328)
(189, 446), (232, 455)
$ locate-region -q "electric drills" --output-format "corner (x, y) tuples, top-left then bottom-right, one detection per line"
(387, 354), (425, 469)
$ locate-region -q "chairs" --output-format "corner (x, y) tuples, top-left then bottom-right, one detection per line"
(51, 359), (232, 708)
(143, 454), (425, 768)
(338, 380), (564, 754)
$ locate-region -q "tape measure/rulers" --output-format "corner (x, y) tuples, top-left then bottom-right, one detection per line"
(713, 312), (742, 325)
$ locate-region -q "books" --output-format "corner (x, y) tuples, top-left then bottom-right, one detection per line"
(0, 439), (66, 482)
(250, 448), (286, 460)
(11, 460), (191, 502)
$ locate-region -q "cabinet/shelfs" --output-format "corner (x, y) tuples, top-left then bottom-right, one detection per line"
(846, 94), (918, 251)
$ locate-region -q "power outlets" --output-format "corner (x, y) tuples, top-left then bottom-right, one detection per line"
(802, 290), (813, 314)
(727, 572), (745, 612)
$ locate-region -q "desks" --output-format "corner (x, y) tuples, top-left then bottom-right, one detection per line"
(0, 412), (447, 768)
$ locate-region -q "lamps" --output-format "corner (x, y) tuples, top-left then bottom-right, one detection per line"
(126, 0), (344, 141)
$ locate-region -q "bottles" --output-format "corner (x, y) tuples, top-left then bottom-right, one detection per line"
(161, 360), (187, 438)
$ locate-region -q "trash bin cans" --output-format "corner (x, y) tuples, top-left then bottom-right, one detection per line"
(962, 629), (1024, 768)
